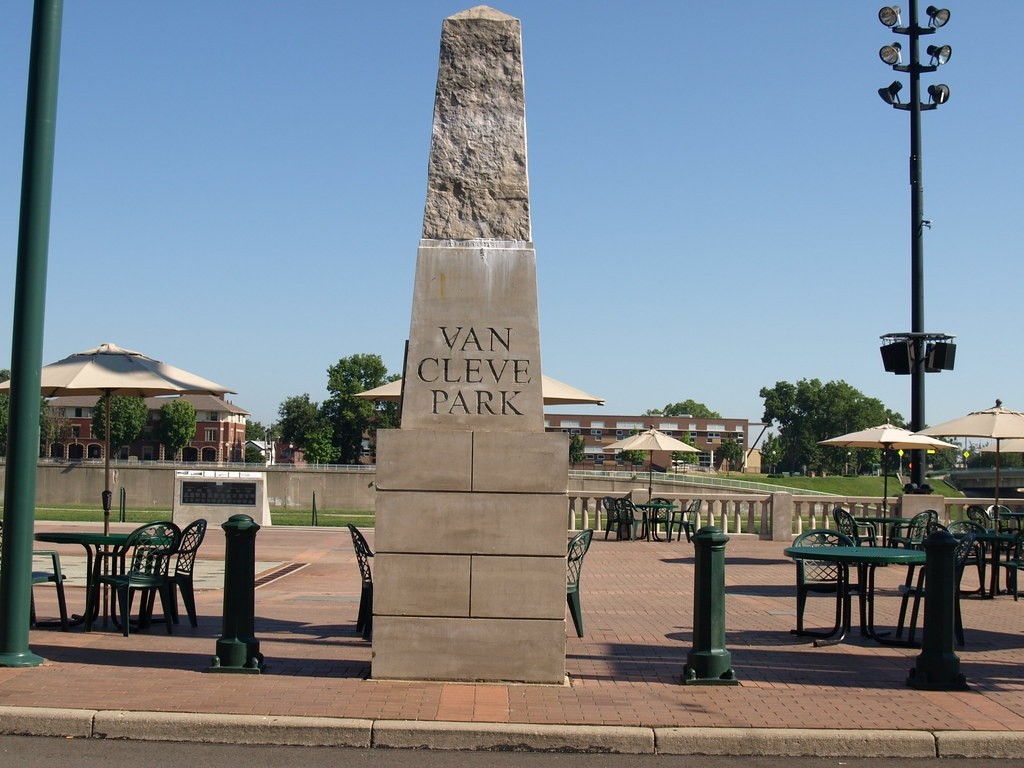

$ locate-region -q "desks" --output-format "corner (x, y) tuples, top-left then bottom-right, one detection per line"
(32, 529), (173, 634)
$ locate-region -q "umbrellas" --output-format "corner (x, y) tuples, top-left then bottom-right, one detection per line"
(816, 417), (959, 450)
(911, 399), (1024, 435)
(978, 429), (1024, 454)
(353, 363), (607, 406)
(0, 342), (239, 397)
(602, 423), (702, 452)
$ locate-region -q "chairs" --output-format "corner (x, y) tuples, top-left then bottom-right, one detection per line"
(564, 528), (593, 638)
(0, 519), (72, 629)
(75, 521), (180, 637)
(603, 491), (1024, 646)
(144, 519), (209, 629)
(347, 522), (374, 643)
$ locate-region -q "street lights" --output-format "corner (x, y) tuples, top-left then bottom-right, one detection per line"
(874, 0), (954, 487)
